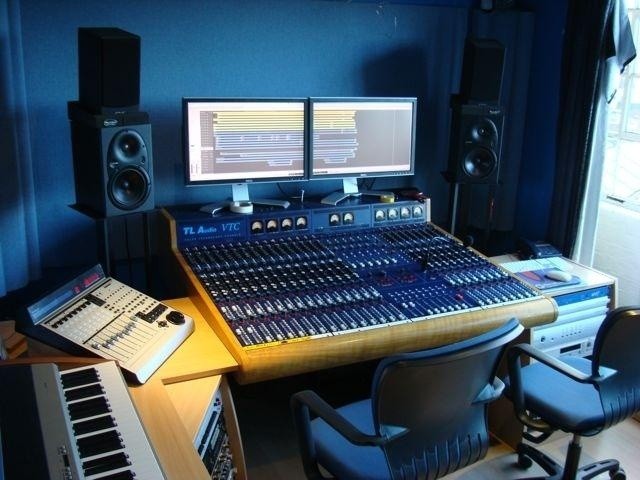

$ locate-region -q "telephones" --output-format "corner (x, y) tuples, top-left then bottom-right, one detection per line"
(518, 237), (563, 260)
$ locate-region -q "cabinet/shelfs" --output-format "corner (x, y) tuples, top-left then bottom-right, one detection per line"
(0, 296), (249, 480)
(486, 253), (619, 453)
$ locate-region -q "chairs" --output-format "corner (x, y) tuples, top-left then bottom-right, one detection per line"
(291, 317), (525, 480)
(500, 304), (640, 480)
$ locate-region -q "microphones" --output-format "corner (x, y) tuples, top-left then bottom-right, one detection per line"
(421, 234), (474, 272)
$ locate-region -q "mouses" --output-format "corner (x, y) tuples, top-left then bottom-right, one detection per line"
(547, 270), (572, 282)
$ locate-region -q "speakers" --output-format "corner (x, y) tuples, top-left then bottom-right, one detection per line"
(78, 27), (141, 114)
(458, 37), (506, 107)
(71, 121), (155, 218)
(447, 110), (504, 184)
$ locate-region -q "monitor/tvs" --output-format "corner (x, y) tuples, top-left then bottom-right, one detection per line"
(309, 97), (418, 206)
(182, 97), (309, 215)
